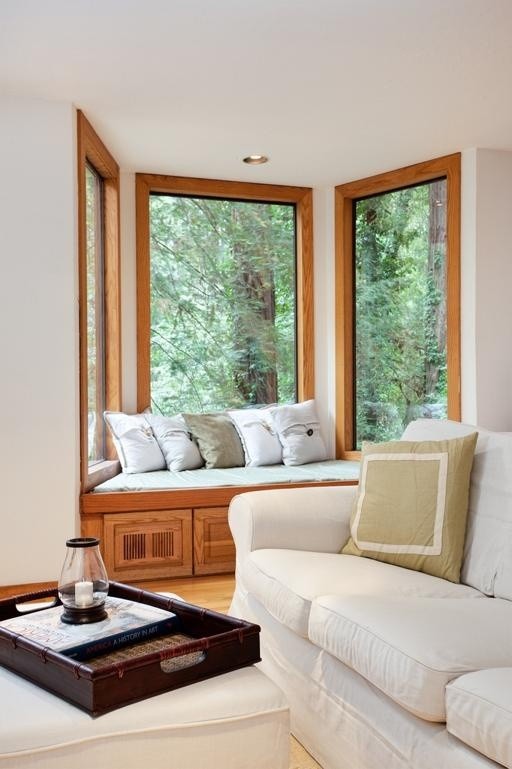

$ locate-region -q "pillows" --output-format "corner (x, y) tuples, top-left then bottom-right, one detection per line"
(338, 431), (485, 583)
(102, 399), (330, 474)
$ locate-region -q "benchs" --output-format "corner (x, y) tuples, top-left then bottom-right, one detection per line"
(79, 458), (365, 583)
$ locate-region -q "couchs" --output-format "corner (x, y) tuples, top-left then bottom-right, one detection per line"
(224, 417), (512, 769)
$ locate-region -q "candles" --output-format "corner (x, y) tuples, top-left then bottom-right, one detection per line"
(75, 576), (93, 606)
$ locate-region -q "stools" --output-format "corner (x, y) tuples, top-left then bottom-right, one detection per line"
(1, 591), (289, 769)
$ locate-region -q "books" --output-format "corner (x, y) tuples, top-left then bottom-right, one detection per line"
(0, 595), (180, 662)
